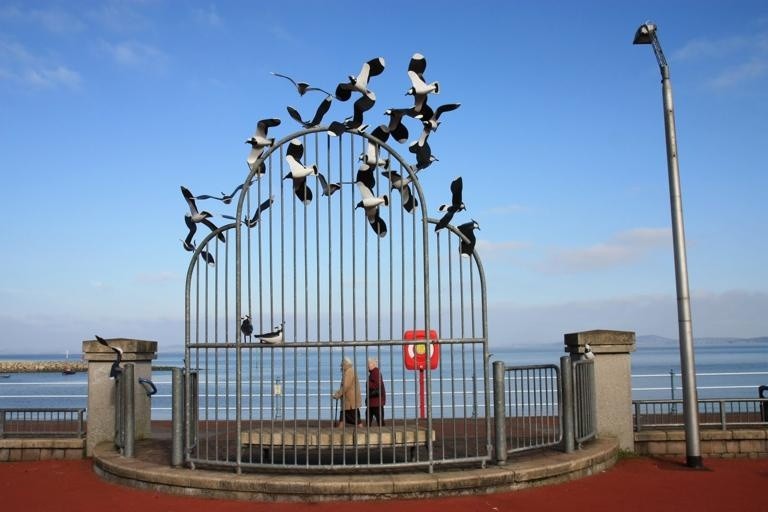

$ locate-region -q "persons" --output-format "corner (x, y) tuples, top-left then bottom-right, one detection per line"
(330, 354), (365, 427)
(361, 356), (387, 426)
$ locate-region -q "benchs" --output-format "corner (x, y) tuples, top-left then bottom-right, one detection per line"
(237, 427), (436, 468)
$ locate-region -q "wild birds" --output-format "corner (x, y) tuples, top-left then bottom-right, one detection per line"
(244, 118), (282, 178)
(182, 215), (215, 265)
(94, 334), (125, 380)
(180, 185), (225, 243)
(457, 218), (481, 260)
(239, 314), (253, 343)
(270, 52), (467, 238)
(189, 178), (259, 204)
(221, 199), (274, 228)
(254, 321), (285, 344)
(578, 343), (596, 361)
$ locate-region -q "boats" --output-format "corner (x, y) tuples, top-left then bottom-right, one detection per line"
(0, 374), (10, 378)
(63, 365), (76, 375)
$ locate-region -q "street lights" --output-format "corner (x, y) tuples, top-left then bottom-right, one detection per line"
(633, 24), (703, 469)
